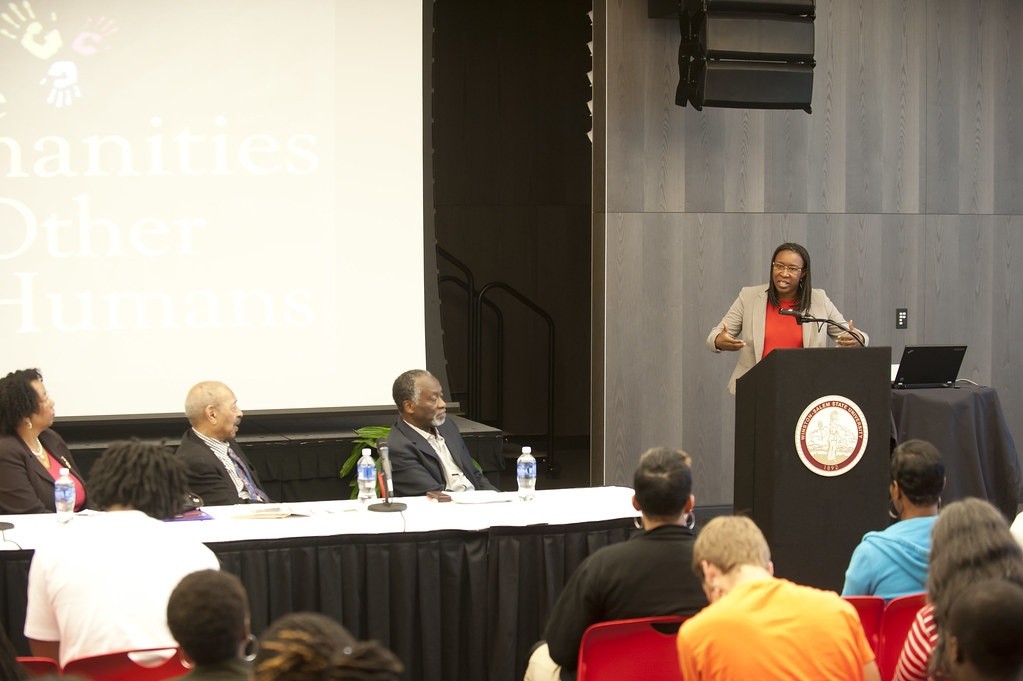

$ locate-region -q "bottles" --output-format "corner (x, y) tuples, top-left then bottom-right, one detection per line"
(357, 449), (377, 506)
(517, 446), (536, 502)
(54, 468), (76, 519)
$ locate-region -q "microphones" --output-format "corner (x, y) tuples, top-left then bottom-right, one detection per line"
(779, 307), (811, 317)
(376, 437), (393, 498)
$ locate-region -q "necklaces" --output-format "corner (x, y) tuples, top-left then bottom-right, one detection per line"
(31, 438), (44, 460)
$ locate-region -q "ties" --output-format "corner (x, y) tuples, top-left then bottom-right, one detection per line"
(227, 447), (269, 503)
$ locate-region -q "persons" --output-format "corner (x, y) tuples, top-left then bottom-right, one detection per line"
(524, 446), (710, 681)
(24, 434), (221, 670)
(677, 515), (882, 681)
(166, 569), (404, 681)
(386, 369), (501, 498)
(177, 381), (280, 506)
(840, 439), (946, 607)
(705, 242), (870, 396)
(891, 498), (1023, 681)
(0, 369), (88, 515)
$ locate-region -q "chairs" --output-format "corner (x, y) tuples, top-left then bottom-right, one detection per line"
(841, 596), (885, 665)
(578, 615), (687, 681)
(880, 592), (930, 681)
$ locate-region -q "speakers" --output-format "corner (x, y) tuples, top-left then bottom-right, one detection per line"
(683, 0), (815, 109)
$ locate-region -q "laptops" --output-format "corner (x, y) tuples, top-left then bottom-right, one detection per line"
(891, 344), (968, 389)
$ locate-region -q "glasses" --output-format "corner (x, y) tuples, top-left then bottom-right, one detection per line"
(772, 260), (805, 275)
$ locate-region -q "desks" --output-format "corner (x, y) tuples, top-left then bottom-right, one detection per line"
(0, 486), (643, 681)
(890, 382), (1023, 528)
